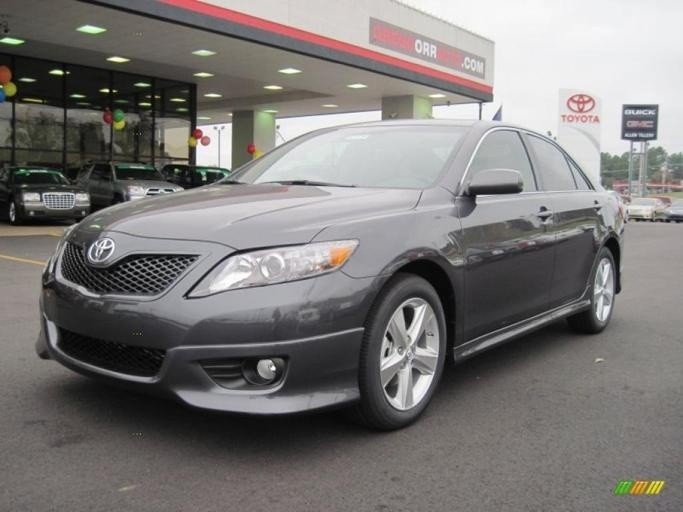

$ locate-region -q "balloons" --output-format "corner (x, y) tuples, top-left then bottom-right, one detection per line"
(0, 64), (11, 84)
(247, 144), (255, 153)
(3, 82), (16, 95)
(188, 137), (198, 147)
(113, 109), (124, 122)
(0, 88), (6, 103)
(193, 128), (203, 139)
(201, 136), (210, 146)
(112, 119), (126, 132)
(102, 113), (113, 124)
(250, 148), (262, 159)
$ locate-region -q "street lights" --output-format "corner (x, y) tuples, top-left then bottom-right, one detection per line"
(213, 125), (225, 168)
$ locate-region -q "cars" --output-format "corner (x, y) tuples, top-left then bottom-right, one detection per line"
(620, 195), (683, 222)
(0, 158), (231, 225)
(32, 117), (628, 432)
